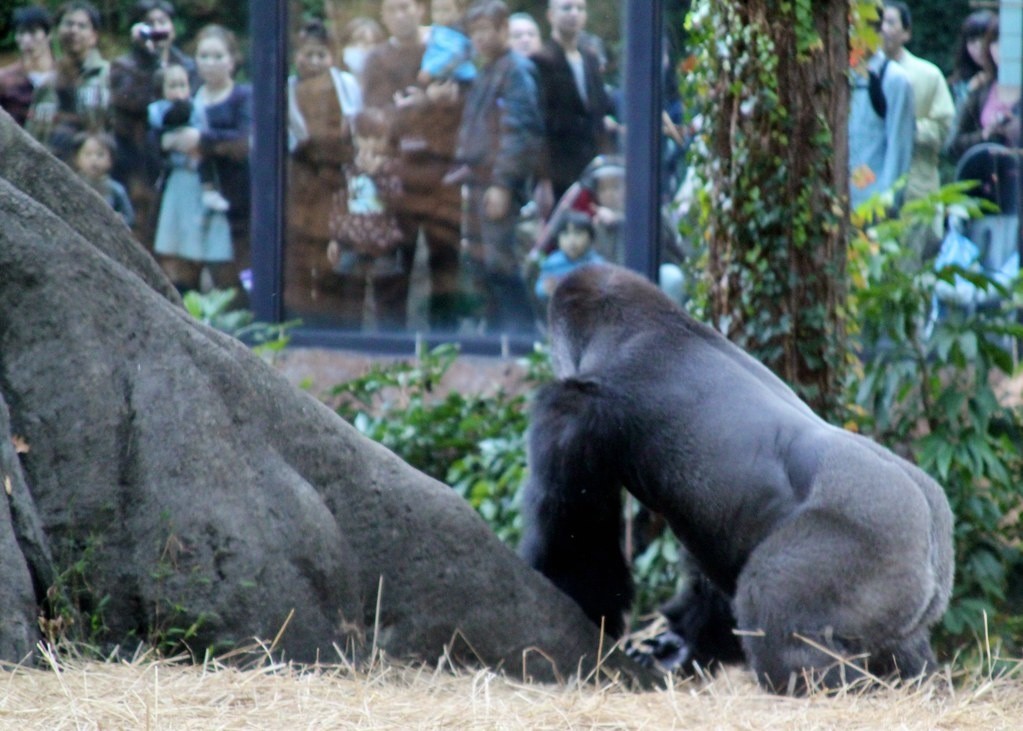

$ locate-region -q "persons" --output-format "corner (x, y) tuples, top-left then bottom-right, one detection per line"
(1, 0), (706, 341)
(848, 0), (913, 227)
(949, 14), (1023, 227)
(940, 9), (1000, 154)
(867, 0), (958, 343)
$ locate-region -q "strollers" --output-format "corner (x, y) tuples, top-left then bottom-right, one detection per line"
(499, 156), (629, 333)
(911, 138), (1023, 369)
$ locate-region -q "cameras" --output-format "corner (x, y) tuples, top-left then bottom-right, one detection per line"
(138, 27), (169, 41)
